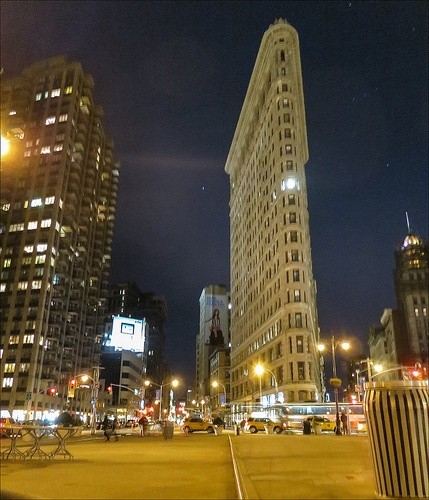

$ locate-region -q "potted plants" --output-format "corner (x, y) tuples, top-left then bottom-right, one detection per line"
(263, 422), (274, 434)
(213, 417), (226, 435)
(55, 412), (84, 438)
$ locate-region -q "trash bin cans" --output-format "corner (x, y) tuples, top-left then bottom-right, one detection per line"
(364, 380), (429, 500)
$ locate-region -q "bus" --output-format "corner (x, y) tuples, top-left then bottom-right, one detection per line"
(247, 406), (275, 434)
(265, 401), (369, 434)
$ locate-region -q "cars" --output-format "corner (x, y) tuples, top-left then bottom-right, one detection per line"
(95, 419), (139, 430)
(0, 417), (22, 436)
(21, 419), (57, 437)
(183, 413), (219, 434)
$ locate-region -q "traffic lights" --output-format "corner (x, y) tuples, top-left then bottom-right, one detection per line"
(70, 378), (76, 388)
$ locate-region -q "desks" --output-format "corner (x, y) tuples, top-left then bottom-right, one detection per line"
(0, 426), (81, 465)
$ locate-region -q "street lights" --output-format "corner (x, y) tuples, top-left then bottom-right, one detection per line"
(212, 381), (226, 430)
(316, 336), (350, 436)
(144, 379), (178, 422)
(255, 365), (278, 402)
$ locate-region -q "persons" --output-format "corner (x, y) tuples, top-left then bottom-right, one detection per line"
(205, 309), (221, 338)
(103, 415), (119, 443)
(139, 415), (148, 437)
(341, 413), (347, 436)
